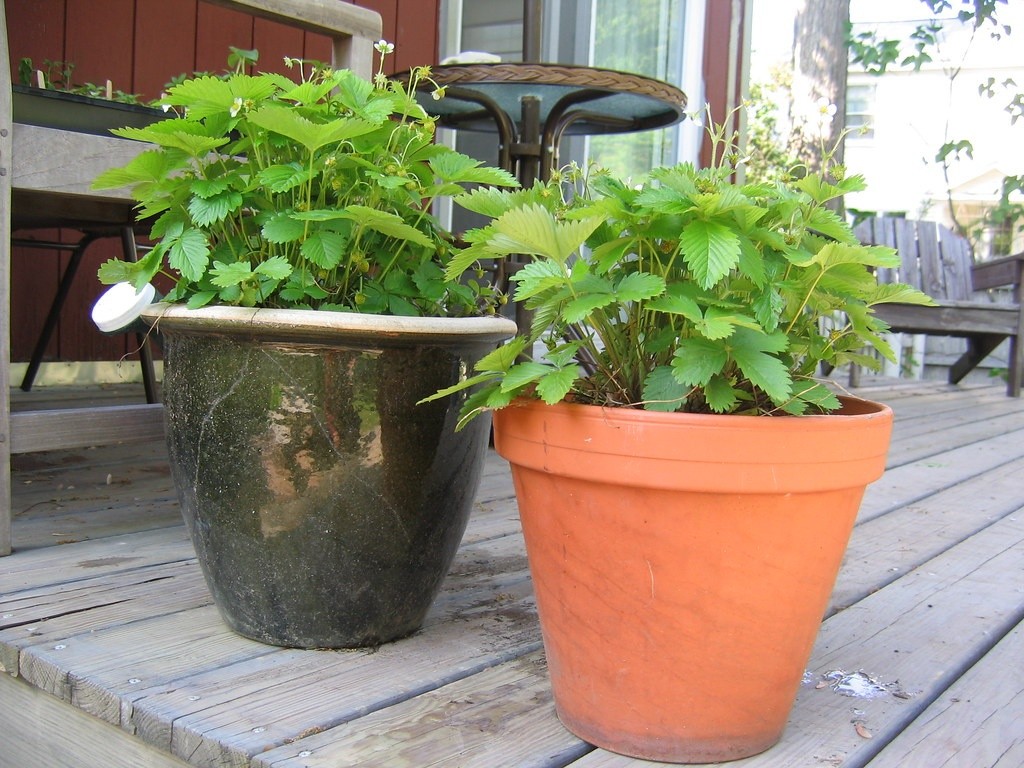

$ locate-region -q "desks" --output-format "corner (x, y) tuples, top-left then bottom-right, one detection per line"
(383, 62), (690, 440)
(10, 122), (260, 407)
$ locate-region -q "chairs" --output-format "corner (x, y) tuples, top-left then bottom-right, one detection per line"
(0, 0), (388, 557)
(820, 216), (1024, 400)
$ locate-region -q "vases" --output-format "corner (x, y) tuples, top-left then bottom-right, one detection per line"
(489, 396), (895, 764)
(144, 306), (517, 652)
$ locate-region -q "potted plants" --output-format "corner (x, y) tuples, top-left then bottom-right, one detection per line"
(10, 56), (243, 156)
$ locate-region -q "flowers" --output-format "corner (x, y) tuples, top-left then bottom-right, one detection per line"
(413, 81), (934, 431)
(97, 39), (515, 317)
